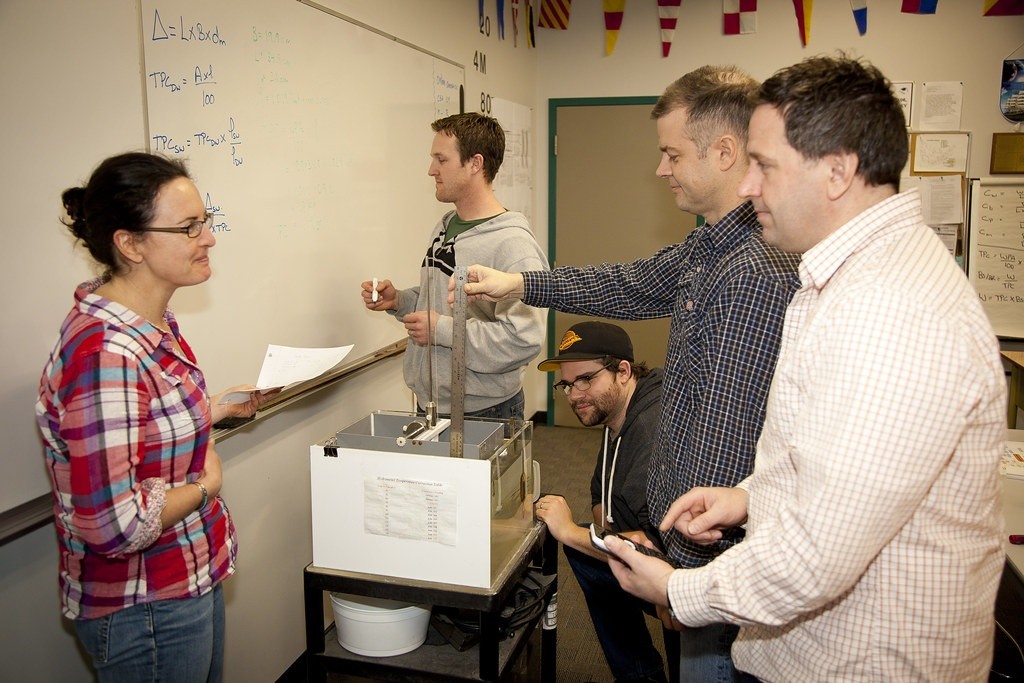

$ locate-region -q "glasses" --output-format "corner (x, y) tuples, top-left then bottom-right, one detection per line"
(127, 213), (213, 238)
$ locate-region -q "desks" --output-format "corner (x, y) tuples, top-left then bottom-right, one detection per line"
(1000, 350), (1024, 429)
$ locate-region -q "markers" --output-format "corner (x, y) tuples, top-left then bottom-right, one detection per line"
(371, 277), (380, 306)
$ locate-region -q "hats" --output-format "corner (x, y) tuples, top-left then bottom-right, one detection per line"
(537, 321), (635, 372)
(552, 361), (615, 395)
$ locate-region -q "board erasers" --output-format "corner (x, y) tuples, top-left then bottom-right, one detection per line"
(214, 412), (256, 429)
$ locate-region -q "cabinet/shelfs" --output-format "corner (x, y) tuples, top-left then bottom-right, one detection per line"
(304, 493), (564, 683)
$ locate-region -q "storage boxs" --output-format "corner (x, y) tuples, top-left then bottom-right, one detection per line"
(329, 592), (433, 657)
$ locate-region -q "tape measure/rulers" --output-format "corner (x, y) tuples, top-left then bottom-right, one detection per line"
(448, 266), (468, 460)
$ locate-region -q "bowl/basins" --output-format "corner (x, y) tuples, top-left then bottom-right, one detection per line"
(329, 590), (433, 657)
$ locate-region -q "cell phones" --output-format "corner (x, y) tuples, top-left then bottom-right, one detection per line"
(589, 524), (684, 570)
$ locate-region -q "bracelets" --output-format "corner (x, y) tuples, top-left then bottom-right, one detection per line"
(190, 481), (208, 512)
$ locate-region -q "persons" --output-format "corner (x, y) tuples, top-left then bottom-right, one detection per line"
(447, 62), (804, 682)
(360, 110), (551, 440)
(602, 42), (1008, 683)
(535, 318), (674, 683)
(34, 151), (286, 683)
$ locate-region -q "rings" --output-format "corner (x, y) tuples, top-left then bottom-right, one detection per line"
(540, 502), (544, 509)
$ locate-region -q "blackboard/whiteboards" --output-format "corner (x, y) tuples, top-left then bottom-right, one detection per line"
(135, 0), (471, 442)
(961, 176), (1024, 343)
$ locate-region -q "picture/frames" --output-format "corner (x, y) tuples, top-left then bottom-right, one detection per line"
(990, 132), (1024, 174)
